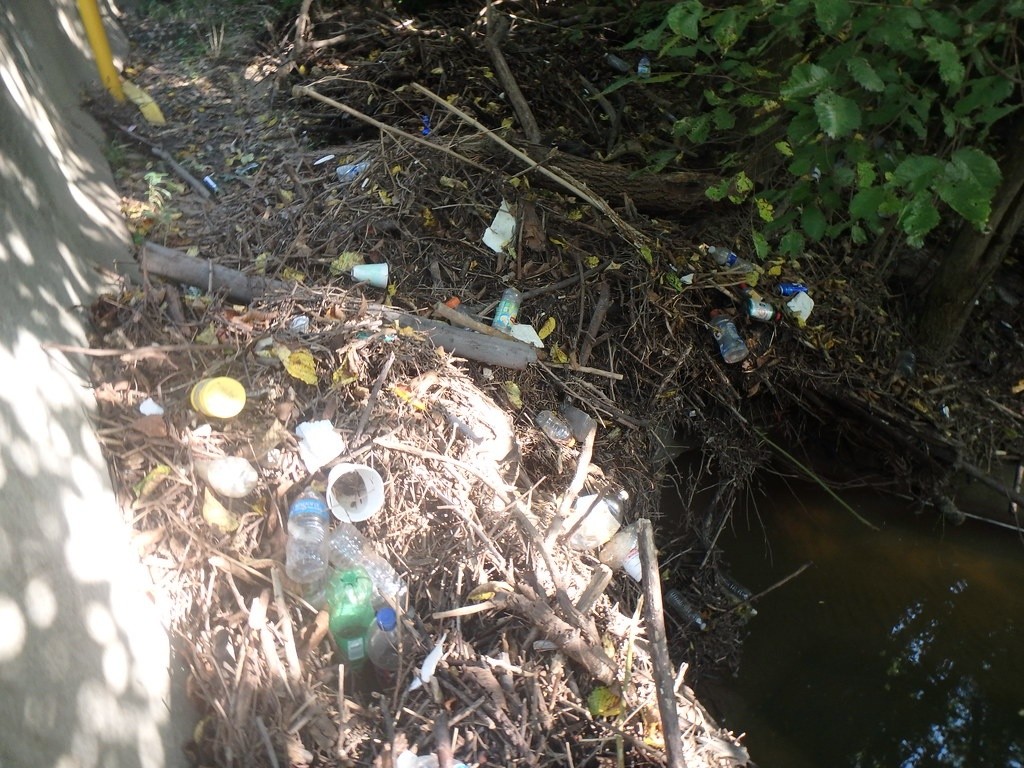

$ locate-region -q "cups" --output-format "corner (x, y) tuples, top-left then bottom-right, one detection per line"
(325, 462), (386, 526)
(350, 262), (389, 289)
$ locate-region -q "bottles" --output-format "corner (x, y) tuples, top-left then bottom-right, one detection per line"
(663, 586), (708, 632)
(603, 52), (631, 73)
(491, 286), (522, 337)
(718, 570), (752, 602)
(337, 160), (371, 182)
(709, 307), (749, 363)
(741, 297), (774, 321)
(708, 245), (753, 279)
(774, 282), (809, 299)
(563, 489), (631, 551)
(328, 521), (416, 626)
(323, 565), (374, 674)
(285, 486), (331, 586)
(363, 606), (412, 681)
(535, 410), (576, 448)
(637, 53), (652, 80)
(622, 545), (648, 584)
